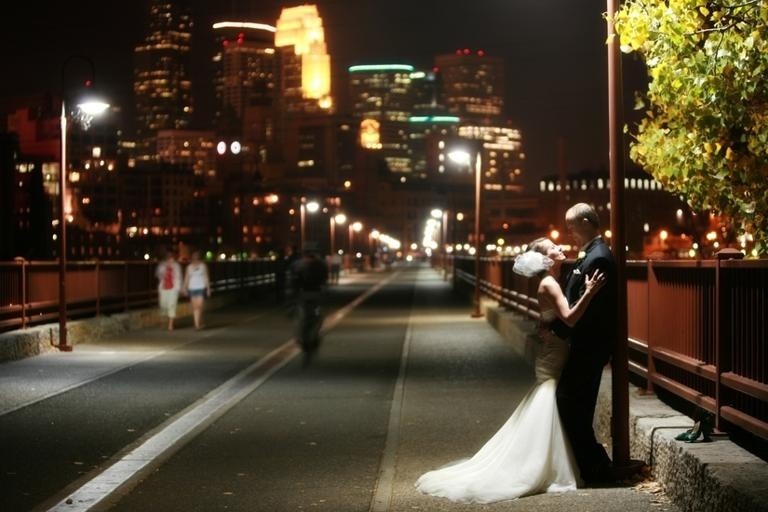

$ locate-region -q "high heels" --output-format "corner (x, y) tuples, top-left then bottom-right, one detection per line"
(675, 415), (715, 444)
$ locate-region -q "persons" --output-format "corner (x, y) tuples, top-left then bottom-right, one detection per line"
(275, 245), (342, 366)
(413, 237), (607, 505)
(184, 251), (212, 330)
(154, 250), (184, 331)
(539, 202), (618, 487)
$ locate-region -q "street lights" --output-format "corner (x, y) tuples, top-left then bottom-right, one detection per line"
(296, 192), (448, 277)
(444, 147), (488, 321)
(214, 137), (244, 259)
(54, 96), (111, 351)
